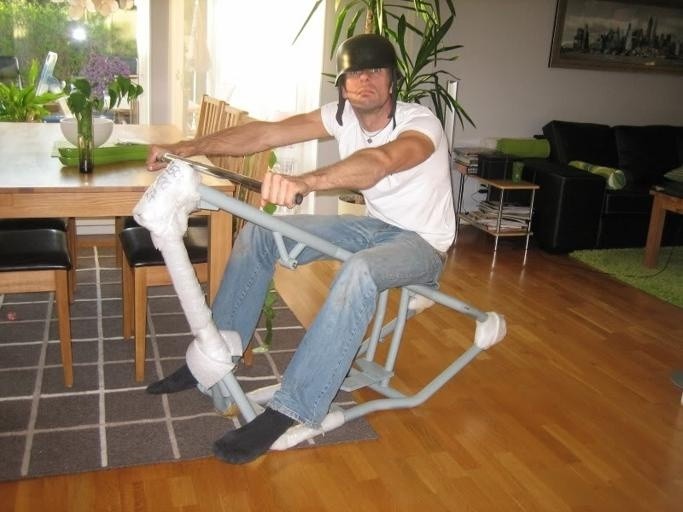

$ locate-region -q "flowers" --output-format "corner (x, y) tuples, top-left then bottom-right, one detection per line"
(62, 50), (143, 166)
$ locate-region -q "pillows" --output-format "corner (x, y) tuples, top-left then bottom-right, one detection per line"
(496, 139), (551, 157)
(651, 166), (683, 200)
(569, 159), (627, 191)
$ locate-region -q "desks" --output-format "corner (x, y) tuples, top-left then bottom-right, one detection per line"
(1, 117), (240, 384)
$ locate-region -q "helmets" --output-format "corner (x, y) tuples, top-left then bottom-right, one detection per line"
(333, 34), (398, 88)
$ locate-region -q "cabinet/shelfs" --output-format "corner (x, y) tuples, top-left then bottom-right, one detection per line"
(454, 173), (541, 269)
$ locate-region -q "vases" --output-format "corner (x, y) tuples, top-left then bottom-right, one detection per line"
(78, 118), (93, 172)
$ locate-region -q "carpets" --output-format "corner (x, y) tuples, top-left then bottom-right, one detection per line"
(2, 245), (378, 484)
(568, 246), (683, 312)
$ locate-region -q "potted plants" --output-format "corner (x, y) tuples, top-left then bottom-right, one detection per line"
(290, 0), (477, 218)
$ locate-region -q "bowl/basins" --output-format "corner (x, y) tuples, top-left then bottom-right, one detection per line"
(61, 117), (114, 149)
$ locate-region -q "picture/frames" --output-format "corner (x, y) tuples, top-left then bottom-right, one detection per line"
(547, 0), (683, 75)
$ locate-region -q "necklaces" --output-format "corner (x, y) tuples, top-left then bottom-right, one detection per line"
(358, 111), (395, 143)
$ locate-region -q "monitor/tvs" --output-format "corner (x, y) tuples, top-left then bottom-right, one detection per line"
(28, 51), (76, 122)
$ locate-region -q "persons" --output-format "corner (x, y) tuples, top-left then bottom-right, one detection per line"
(143, 35), (457, 465)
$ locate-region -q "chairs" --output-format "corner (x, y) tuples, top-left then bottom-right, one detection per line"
(1, 213), (84, 388)
(121, 95), (273, 383)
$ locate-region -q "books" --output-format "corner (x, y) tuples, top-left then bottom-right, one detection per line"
(460, 199), (532, 234)
(452, 145), (504, 176)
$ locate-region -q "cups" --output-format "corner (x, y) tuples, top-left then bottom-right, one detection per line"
(511, 162), (524, 185)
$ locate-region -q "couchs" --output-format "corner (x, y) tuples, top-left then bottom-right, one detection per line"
(490, 119), (683, 255)
